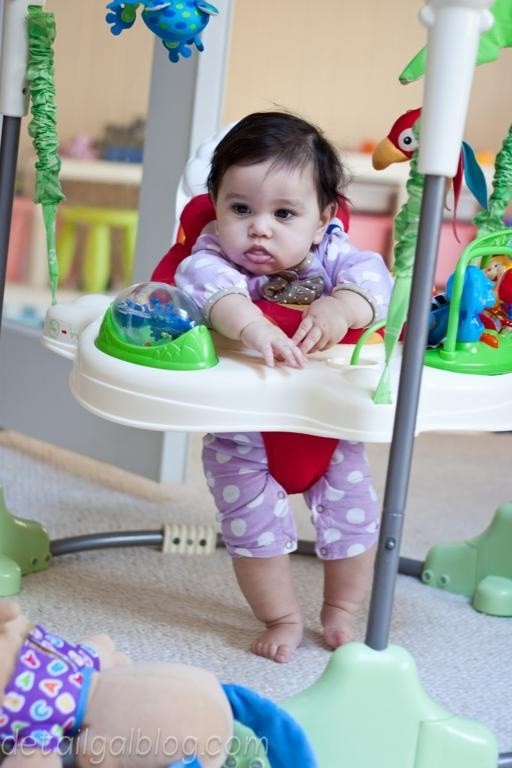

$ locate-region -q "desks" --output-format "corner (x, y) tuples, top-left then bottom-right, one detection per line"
(30, 154), (496, 295)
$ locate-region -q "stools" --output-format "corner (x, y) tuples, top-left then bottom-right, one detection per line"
(56, 207), (138, 292)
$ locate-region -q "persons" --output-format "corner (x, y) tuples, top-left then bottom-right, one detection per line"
(172, 110), (395, 665)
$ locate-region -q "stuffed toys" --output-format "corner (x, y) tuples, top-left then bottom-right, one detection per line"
(1, 596), (317, 768)
